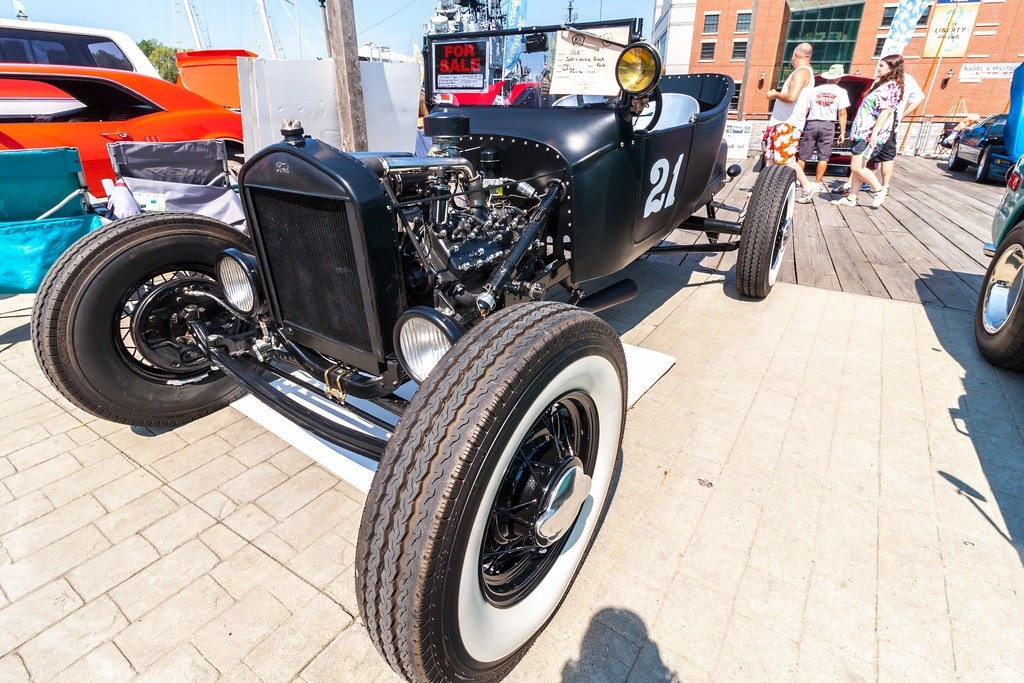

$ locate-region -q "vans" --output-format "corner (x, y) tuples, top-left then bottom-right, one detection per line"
(0, 19), (163, 117)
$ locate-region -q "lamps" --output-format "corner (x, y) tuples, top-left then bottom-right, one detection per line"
(943, 69), (954, 85)
(761, 71), (766, 85)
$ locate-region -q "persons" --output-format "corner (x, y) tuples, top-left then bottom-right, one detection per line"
(763, 40), (818, 205)
(943, 113), (981, 149)
(795, 63), (851, 192)
(830, 55), (926, 209)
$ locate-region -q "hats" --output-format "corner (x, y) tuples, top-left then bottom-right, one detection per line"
(821, 64), (849, 80)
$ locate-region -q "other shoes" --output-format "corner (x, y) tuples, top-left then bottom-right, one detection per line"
(795, 179), (802, 190)
(815, 182), (824, 192)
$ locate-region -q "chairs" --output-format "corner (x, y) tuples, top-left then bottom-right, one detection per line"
(933, 122), (960, 160)
(0, 138), (252, 313)
(552, 94), (608, 106)
(632, 93), (701, 133)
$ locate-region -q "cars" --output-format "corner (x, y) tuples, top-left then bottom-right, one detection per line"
(794, 73), (875, 177)
(0, 62), (245, 199)
(28, 2), (799, 683)
(947, 113), (1016, 184)
(973, 151), (1024, 373)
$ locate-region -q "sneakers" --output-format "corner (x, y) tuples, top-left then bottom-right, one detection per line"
(887, 188), (890, 195)
(831, 196), (856, 207)
(859, 184), (870, 190)
(831, 183), (851, 193)
(871, 187), (887, 208)
(796, 183), (819, 203)
